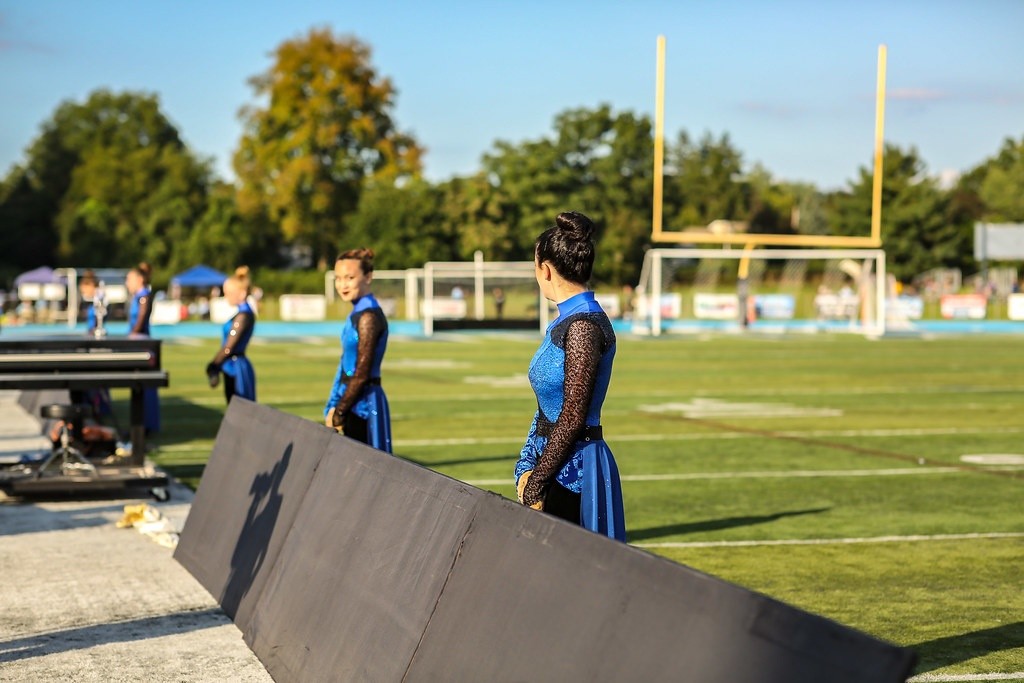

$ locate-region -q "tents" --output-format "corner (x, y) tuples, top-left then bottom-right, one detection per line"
(170, 265), (227, 301)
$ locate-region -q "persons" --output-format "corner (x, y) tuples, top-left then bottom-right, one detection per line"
(322, 246), (393, 458)
(514, 212), (626, 544)
(69, 261), (162, 452)
(205, 265), (256, 406)
(812, 273), (1024, 321)
